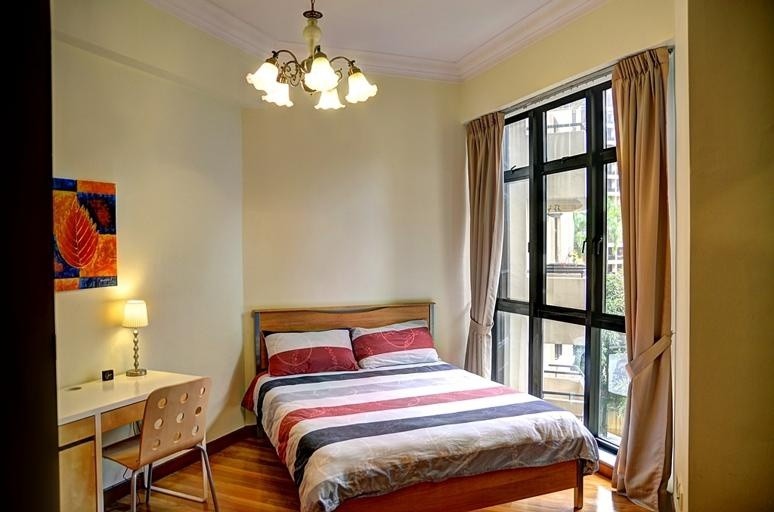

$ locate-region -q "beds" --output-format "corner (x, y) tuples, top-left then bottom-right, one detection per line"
(238, 301), (597, 511)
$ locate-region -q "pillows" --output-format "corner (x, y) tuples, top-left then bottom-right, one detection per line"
(349, 317), (439, 369)
(263, 327), (361, 376)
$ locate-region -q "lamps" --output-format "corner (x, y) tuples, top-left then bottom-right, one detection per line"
(120, 298), (148, 377)
(245, 1), (378, 112)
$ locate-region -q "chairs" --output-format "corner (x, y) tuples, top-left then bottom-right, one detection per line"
(101, 373), (221, 511)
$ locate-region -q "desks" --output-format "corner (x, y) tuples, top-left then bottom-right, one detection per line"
(56, 366), (211, 510)
